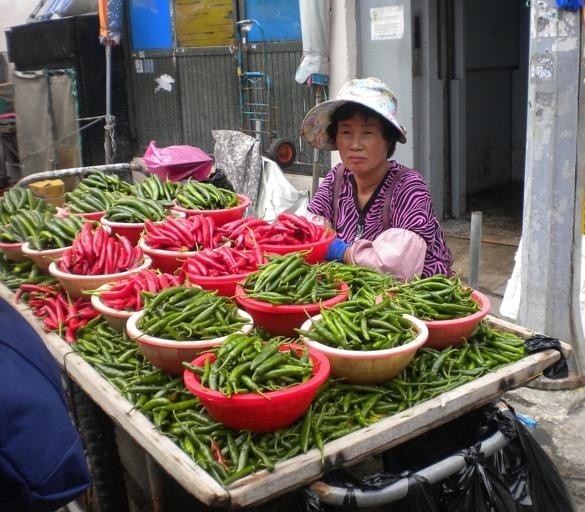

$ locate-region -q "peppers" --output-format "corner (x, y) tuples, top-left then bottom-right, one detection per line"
(1, 170), (527, 485)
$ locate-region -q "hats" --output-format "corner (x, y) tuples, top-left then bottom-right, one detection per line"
(301, 77), (407, 152)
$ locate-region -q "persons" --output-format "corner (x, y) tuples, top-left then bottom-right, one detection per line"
(301, 77), (456, 285)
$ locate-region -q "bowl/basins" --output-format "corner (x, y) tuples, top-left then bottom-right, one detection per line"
(181, 259), (272, 296)
(48, 253), (152, 296)
(22, 236), (74, 271)
(0, 239), (23, 263)
(232, 277), (350, 331)
(60, 206), (105, 220)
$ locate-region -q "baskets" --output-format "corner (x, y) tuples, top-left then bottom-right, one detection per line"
(21, 225), (112, 274)
(1, 243), (28, 263)
(1, 182), (492, 430)
(48, 254), (152, 303)
(184, 250), (269, 297)
(235, 278), (349, 338)
(72, 210), (106, 223)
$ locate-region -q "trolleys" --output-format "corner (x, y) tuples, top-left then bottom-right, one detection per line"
(235, 19), (296, 169)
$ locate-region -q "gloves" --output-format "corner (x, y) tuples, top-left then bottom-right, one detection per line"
(326, 238), (352, 263)
